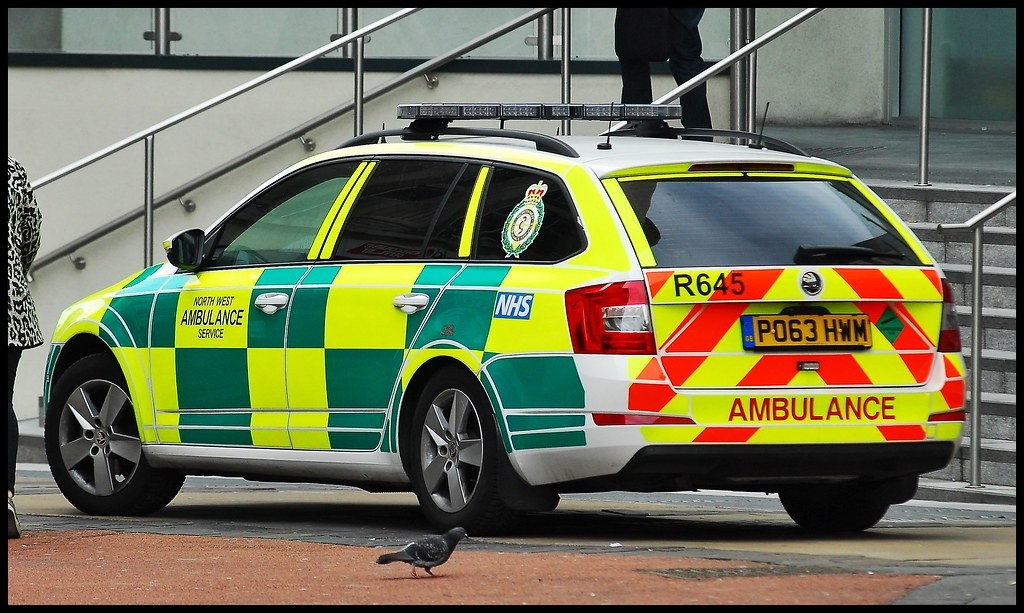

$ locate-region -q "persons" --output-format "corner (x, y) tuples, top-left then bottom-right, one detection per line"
(614, 7), (716, 142)
(7, 157), (43, 539)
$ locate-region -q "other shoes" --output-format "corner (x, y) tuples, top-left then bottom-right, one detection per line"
(8, 496), (22, 539)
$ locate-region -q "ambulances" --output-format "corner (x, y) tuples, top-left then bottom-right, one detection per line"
(39, 102), (968, 538)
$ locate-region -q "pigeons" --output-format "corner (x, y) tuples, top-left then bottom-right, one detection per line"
(376, 527), (470, 579)
